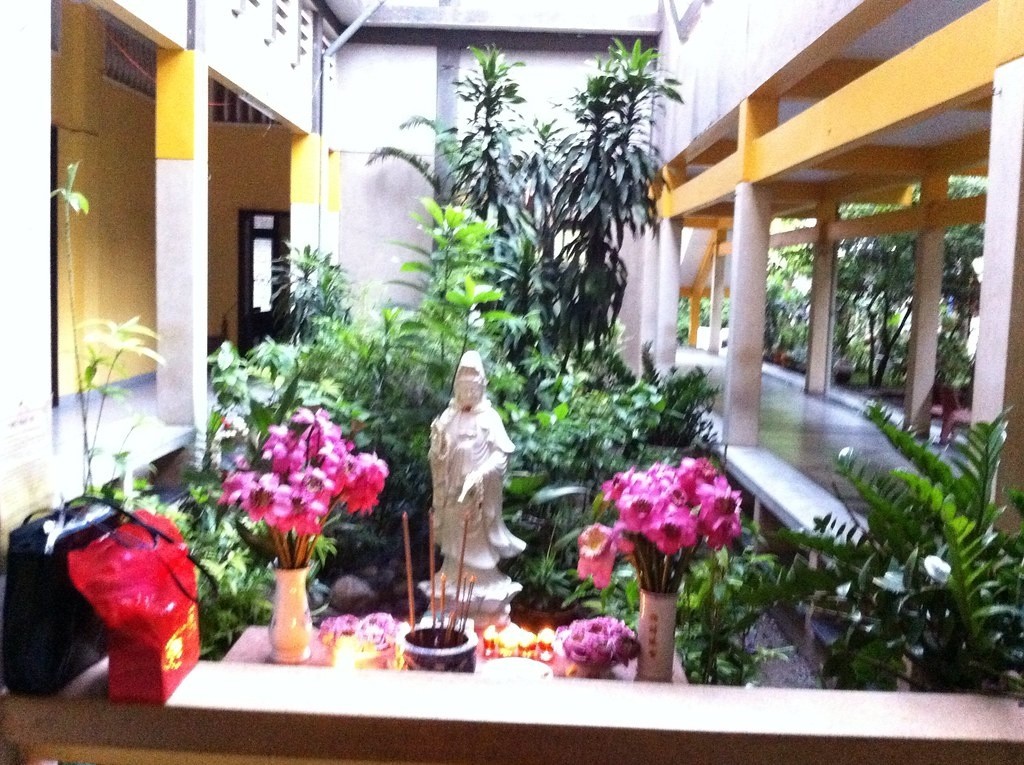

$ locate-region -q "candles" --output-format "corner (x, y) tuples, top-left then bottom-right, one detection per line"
(537, 627), (555, 662)
(498, 622), (518, 657)
(482, 625), (497, 658)
(518, 632), (537, 660)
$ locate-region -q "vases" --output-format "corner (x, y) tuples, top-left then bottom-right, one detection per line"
(634, 590), (680, 681)
(268, 567), (314, 662)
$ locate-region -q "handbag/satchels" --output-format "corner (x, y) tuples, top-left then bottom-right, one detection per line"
(3, 501), (124, 697)
(69, 509), (200, 706)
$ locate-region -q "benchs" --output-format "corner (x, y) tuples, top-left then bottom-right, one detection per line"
(66, 423), (196, 503)
(719, 444), (870, 546)
(0, 661), (1024, 765)
(694, 405), (723, 450)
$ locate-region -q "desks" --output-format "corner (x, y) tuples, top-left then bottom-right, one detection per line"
(220, 624), (688, 685)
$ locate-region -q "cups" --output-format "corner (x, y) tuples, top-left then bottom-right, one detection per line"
(483, 630), (553, 661)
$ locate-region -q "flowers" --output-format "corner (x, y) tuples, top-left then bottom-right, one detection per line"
(577, 458), (743, 593)
(319, 612), (396, 656)
(563, 616), (637, 680)
(219, 408), (389, 566)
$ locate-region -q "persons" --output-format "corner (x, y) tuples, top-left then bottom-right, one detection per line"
(427, 349), (527, 573)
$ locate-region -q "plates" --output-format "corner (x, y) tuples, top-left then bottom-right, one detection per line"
(476, 657), (554, 681)
